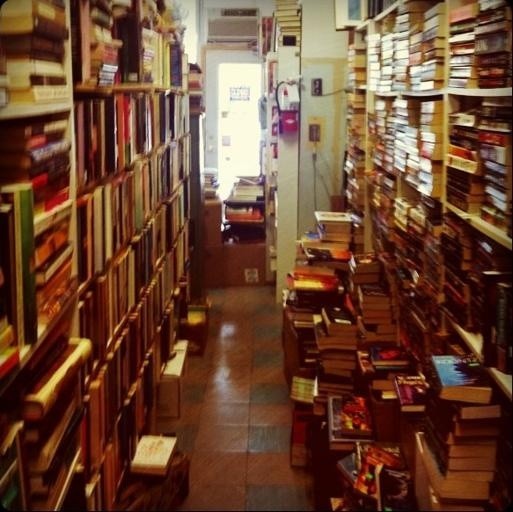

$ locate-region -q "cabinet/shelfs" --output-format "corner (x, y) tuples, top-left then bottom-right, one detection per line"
(0, 0), (200, 512)
(345, 1), (511, 437)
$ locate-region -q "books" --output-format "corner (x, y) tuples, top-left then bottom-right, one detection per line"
(225, 0), (512, 511)
(2, 0), (204, 507)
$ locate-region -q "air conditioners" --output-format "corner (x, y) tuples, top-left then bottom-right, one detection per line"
(207, 6), (261, 42)
